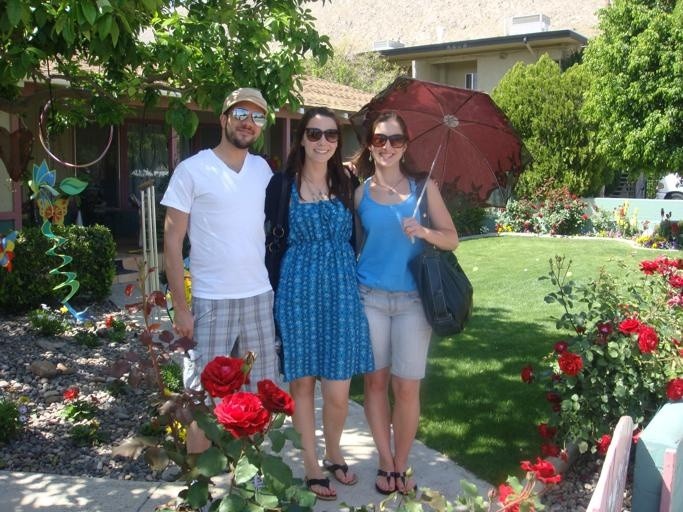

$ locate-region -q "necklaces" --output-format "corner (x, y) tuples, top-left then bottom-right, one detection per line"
(372, 178), (407, 201)
(301, 174), (333, 203)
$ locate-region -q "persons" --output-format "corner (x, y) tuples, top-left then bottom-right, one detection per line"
(156, 85), (281, 512)
(348, 111), (461, 497)
(266, 105), (373, 504)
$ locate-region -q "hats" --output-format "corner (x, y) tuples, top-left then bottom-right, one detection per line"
(222, 86), (270, 116)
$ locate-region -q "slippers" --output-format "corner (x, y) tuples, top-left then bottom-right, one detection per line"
(374, 468), (395, 495)
(323, 458), (360, 485)
(304, 474), (338, 502)
(396, 472), (417, 495)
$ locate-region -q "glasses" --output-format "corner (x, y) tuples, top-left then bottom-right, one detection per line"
(369, 132), (410, 150)
(223, 107), (266, 128)
(302, 126), (341, 144)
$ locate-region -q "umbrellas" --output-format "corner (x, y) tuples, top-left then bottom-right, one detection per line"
(346, 76), (531, 238)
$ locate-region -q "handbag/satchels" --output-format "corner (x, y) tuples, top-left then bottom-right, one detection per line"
(406, 177), (475, 338)
